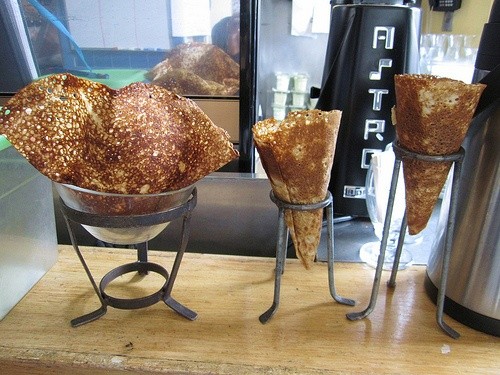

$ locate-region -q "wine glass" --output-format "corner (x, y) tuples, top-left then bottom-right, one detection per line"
(419, 32), (478, 85)
(359, 145), (412, 270)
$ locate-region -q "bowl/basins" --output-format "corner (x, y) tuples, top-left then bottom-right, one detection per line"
(52, 181), (200, 245)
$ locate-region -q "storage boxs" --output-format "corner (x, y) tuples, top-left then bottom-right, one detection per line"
(0, 105), (58, 322)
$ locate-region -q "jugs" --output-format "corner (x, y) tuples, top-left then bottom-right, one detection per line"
(424, 0), (500, 337)
